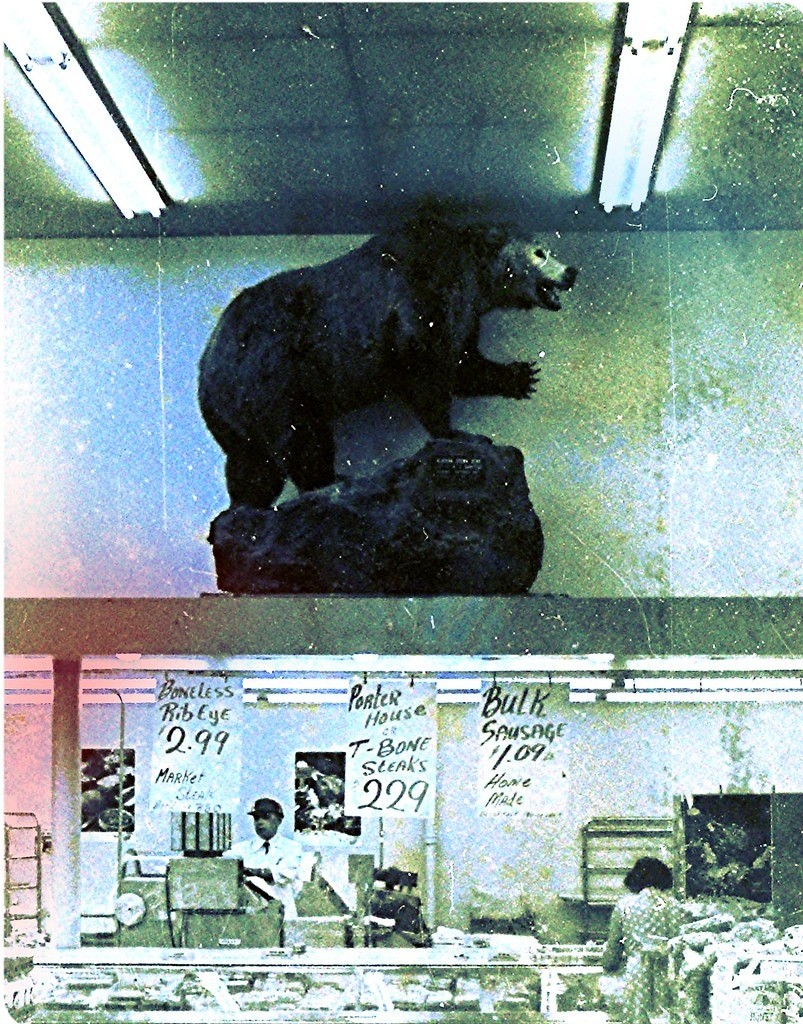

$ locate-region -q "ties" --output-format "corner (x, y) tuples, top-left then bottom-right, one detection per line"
(263, 842), (270, 854)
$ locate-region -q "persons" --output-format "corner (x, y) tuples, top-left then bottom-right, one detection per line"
(601, 857), (692, 1024)
(222, 799), (303, 922)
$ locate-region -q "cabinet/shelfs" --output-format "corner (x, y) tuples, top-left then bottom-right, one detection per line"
(4, 811), (43, 940)
(578, 814), (688, 945)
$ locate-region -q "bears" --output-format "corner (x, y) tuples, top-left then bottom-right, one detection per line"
(193, 210), (579, 515)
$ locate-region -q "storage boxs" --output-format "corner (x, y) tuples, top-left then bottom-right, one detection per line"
(114, 856), (350, 949)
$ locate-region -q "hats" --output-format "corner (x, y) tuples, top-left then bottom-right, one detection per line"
(247, 798), (283, 818)
